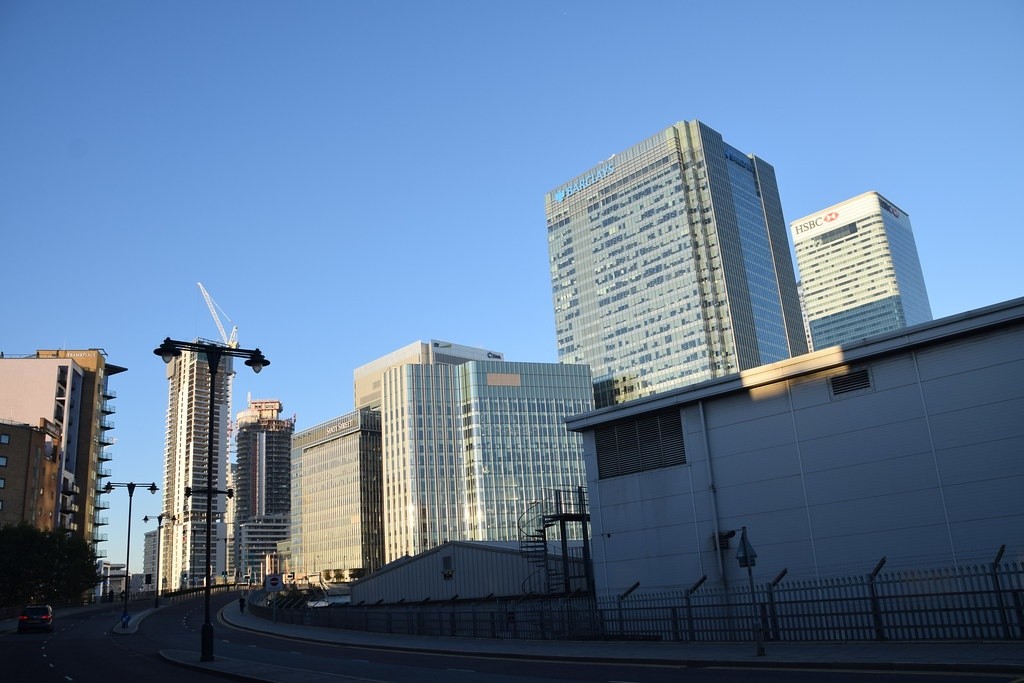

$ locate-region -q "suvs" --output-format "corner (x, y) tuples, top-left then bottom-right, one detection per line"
(17, 605), (56, 634)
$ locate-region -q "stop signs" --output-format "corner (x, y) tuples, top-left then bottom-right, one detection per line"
(265, 573), (283, 593)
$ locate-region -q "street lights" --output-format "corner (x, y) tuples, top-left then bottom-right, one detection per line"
(239, 543), (255, 574)
(142, 513), (178, 608)
(155, 337), (272, 663)
(215, 537), (235, 584)
(182, 527), (207, 595)
(102, 480), (160, 629)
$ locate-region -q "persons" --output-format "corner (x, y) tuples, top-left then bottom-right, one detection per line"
(240, 598), (245, 611)
(109, 589), (114, 602)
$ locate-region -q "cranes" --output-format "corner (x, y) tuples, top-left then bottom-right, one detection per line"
(195, 280), (242, 348)
